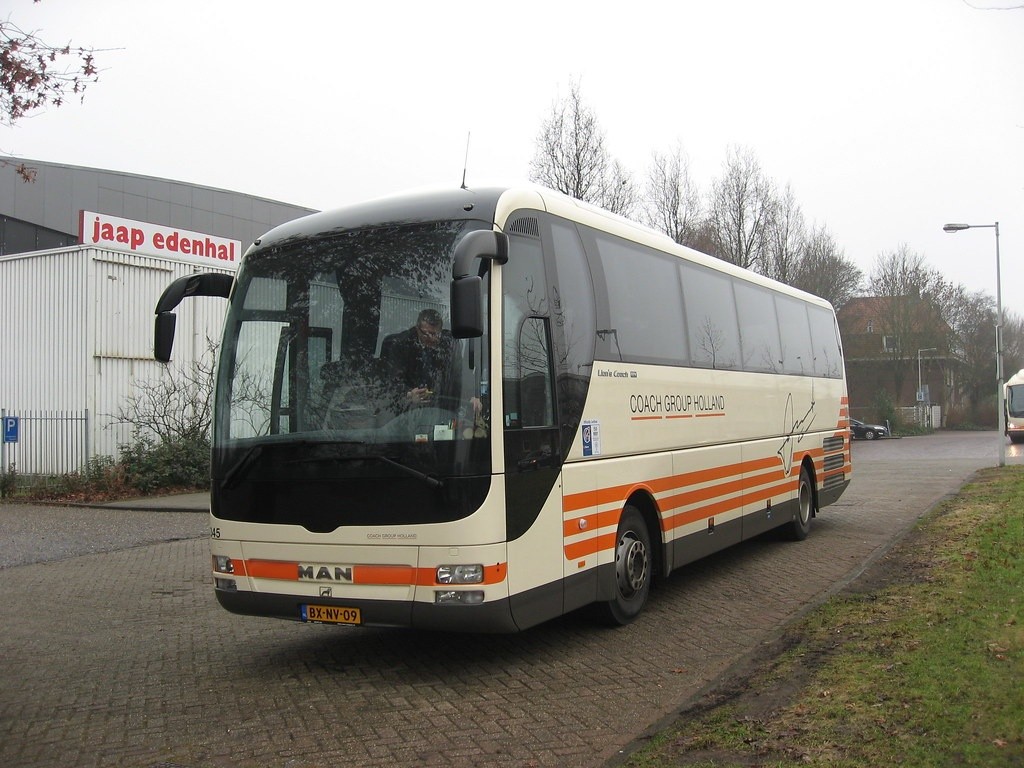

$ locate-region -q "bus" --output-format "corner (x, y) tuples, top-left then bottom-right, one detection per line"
(153, 181), (851, 636)
(1003, 369), (1023, 444)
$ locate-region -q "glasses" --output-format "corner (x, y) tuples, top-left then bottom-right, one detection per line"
(417, 324), (442, 339)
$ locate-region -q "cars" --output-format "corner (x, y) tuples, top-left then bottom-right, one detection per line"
(850, 430), (855, 441)
(849, 418), (889, 440)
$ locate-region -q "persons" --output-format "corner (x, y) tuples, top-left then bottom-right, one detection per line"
(329, 310), (491, 428)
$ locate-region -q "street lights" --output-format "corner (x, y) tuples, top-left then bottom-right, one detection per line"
(944, 222), (1006, 468)
(918, 348), (937, 427)
